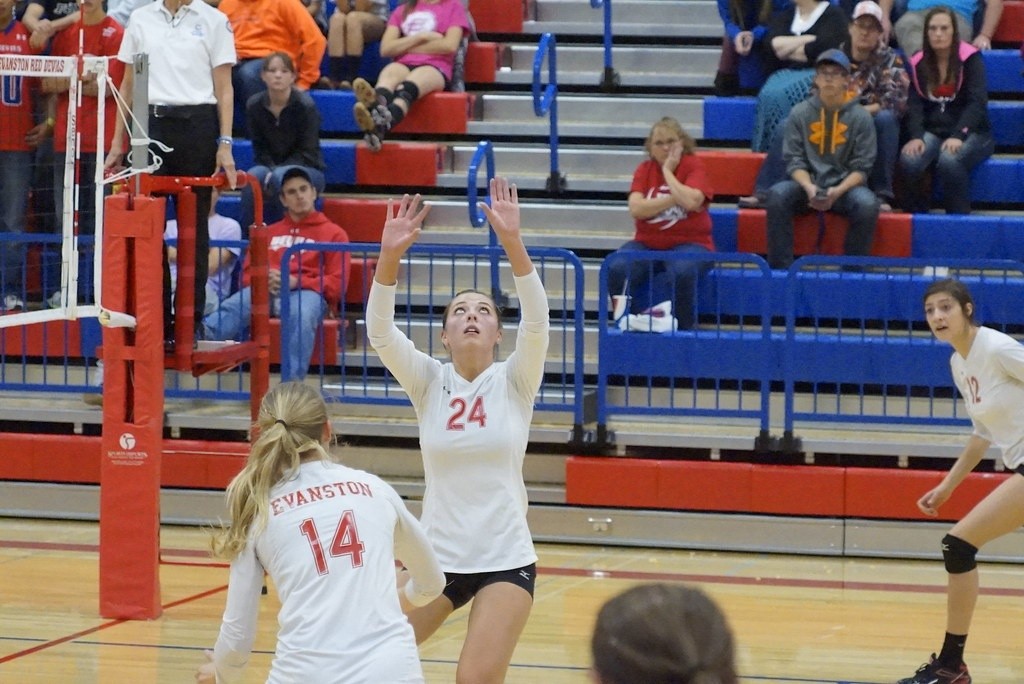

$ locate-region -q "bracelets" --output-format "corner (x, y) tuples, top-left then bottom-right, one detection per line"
(45, 118), (56, 128)
(219, 136), (234, 143)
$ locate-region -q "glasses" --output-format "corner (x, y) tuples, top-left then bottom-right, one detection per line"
(818, 69), (845, 80)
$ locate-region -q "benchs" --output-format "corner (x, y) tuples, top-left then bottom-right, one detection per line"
(0, 0), (536, 369)
(607, 0), (1024, 389)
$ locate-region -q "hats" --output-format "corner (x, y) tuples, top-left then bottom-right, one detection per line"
(850, 0), (884, 30)
(280, 167), (312, 184)
(815, 47), (854, 75)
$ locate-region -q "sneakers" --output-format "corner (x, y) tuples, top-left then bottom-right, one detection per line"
(899, 653), (973, 683)
(3, 295), (23, 311)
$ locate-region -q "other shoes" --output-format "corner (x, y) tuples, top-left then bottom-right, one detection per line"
(352, 101), (388, 152)
(319, 76), (336, 90)
(47, 291), (62, 308)
(740, 192), (766, 210)
(877, 197), (894, 217)
(354, 76), (384, 111)
(340, 79), (352, 92)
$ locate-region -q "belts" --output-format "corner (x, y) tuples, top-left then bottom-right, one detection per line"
(149, 104), (214, 121)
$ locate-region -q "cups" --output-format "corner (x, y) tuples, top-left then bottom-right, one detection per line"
(613, 295), (633, 328)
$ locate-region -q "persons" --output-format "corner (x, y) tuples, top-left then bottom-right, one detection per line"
(196, 380), (447, 684)
(365, 177), (551, 684)
(894, 278), (1024, 684)
(716, 0), (1004, 216)
(0, 0), (476, 401)
(605, 117), (713, 313)
(765, 49), (879, 274)
(591, 581), (739, 684)
